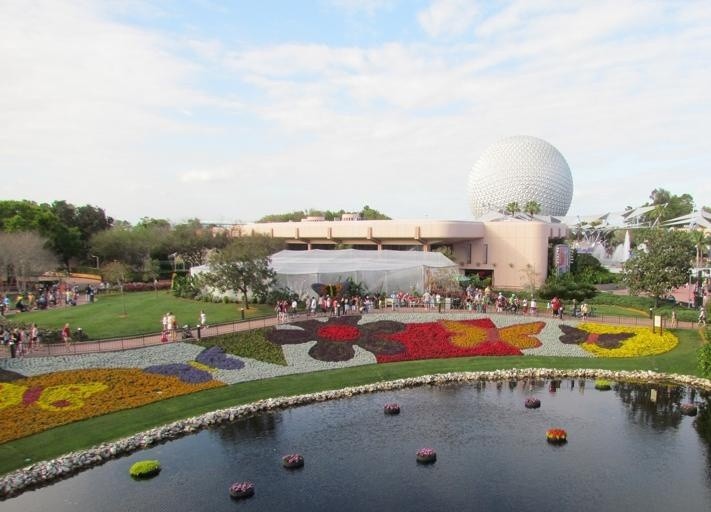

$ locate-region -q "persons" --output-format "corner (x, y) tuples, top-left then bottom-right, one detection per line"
(274, 284), (537, 324)
(162, 309), (209, 340)
(0, 280), (112, 357)
(549, 295), (589, 321)
(671, 276), (711, 328)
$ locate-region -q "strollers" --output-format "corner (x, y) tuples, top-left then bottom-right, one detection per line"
(178, 325), (194, 340)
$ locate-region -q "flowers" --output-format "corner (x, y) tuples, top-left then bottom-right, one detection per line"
(525, 399), (540, 407)
(415, 447), (436, 457)
(230, 481), (255, 493)
(283, 454), (303, 464)
(385, 403), (400, 411)
(546, 429), (567, 441)
